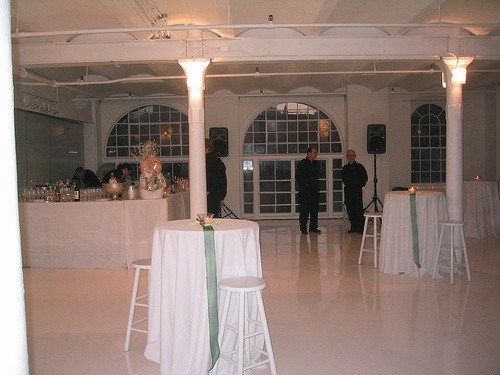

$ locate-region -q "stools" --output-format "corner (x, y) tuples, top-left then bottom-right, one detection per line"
(430, 220), (472, 285)
(212, 276), (278, 375)
(123, 258), (152, 352)
(358, 213), (382, 269)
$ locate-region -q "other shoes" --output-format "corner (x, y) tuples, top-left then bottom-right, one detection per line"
(347, 230), (357, 233)
(309, 229), (321, 233)
(302, 231), (307, 234)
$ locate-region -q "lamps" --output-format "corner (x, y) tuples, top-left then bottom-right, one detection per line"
(449, 52), (466, 84)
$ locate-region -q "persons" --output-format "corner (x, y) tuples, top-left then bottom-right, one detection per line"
(71, 166), (102, 190)
(204, 138), (227, 218)
(102, 163), (132, 197)
(295, 147), (322, 233)
(341, 150), (368, 234)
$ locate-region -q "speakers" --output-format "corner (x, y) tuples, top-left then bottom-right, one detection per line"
(366, 124), (386, 154)
(209, 127), (228, 157)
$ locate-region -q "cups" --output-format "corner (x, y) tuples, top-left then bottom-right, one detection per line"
(126, 185), (140, 199)
(475, 176), (481, 181)
(197, 213), (214, 226)
(431, 186), (437, 190)
(409, 186), (417, 195)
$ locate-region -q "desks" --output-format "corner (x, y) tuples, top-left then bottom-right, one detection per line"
(463, 181), (500, 240)
(18, 189), (191, 269)
(144, 218), (264, 375)
(377, 189), (459, 277)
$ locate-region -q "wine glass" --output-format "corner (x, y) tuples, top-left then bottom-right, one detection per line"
(21, 187), (107, 202)
(166, 177), (189, 195)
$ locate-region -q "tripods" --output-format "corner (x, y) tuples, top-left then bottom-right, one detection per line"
(363, 154), (384, 222)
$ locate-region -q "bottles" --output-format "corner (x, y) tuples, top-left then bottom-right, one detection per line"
(109, 172), (117, 184)
(22, 177), (81, 204)
